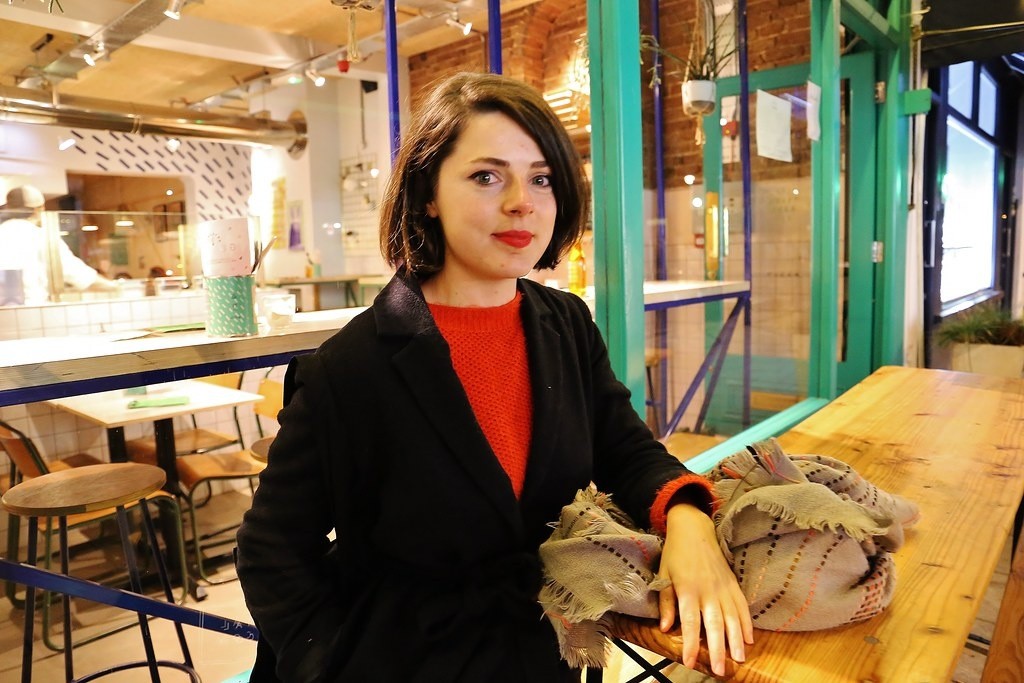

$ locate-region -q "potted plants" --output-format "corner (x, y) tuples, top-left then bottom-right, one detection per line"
(644, 5), (739, 116)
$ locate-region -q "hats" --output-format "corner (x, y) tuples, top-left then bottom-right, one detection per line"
(7, 185), (45, 208)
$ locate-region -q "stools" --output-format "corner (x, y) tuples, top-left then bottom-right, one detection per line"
(0, 463), (203, 683)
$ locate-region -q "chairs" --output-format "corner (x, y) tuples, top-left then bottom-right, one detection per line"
(1, 346), (670, 610)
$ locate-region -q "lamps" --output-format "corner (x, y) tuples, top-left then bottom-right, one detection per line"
(305, 70), (326, 87)
(83, 49), (111, 67)
(446, 19), (473, 35)
(164, 0), (185, 20)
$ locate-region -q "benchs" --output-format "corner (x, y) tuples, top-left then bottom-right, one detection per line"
(980, 523), (1024, 683)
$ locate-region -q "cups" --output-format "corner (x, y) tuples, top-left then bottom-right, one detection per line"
(255, 286), (288, 326)
(264, 293), (296, 332)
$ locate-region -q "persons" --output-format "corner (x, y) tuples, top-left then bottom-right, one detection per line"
(0, 186), (117, 305)
(233, 72), (754, 683)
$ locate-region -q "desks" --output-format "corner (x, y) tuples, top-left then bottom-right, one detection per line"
(46, 379), (266, 602)
(582, 365), (1024, 683)
(0, 280), (752, 641)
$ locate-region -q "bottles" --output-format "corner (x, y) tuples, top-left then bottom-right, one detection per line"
(567, 238), (586, 299)
